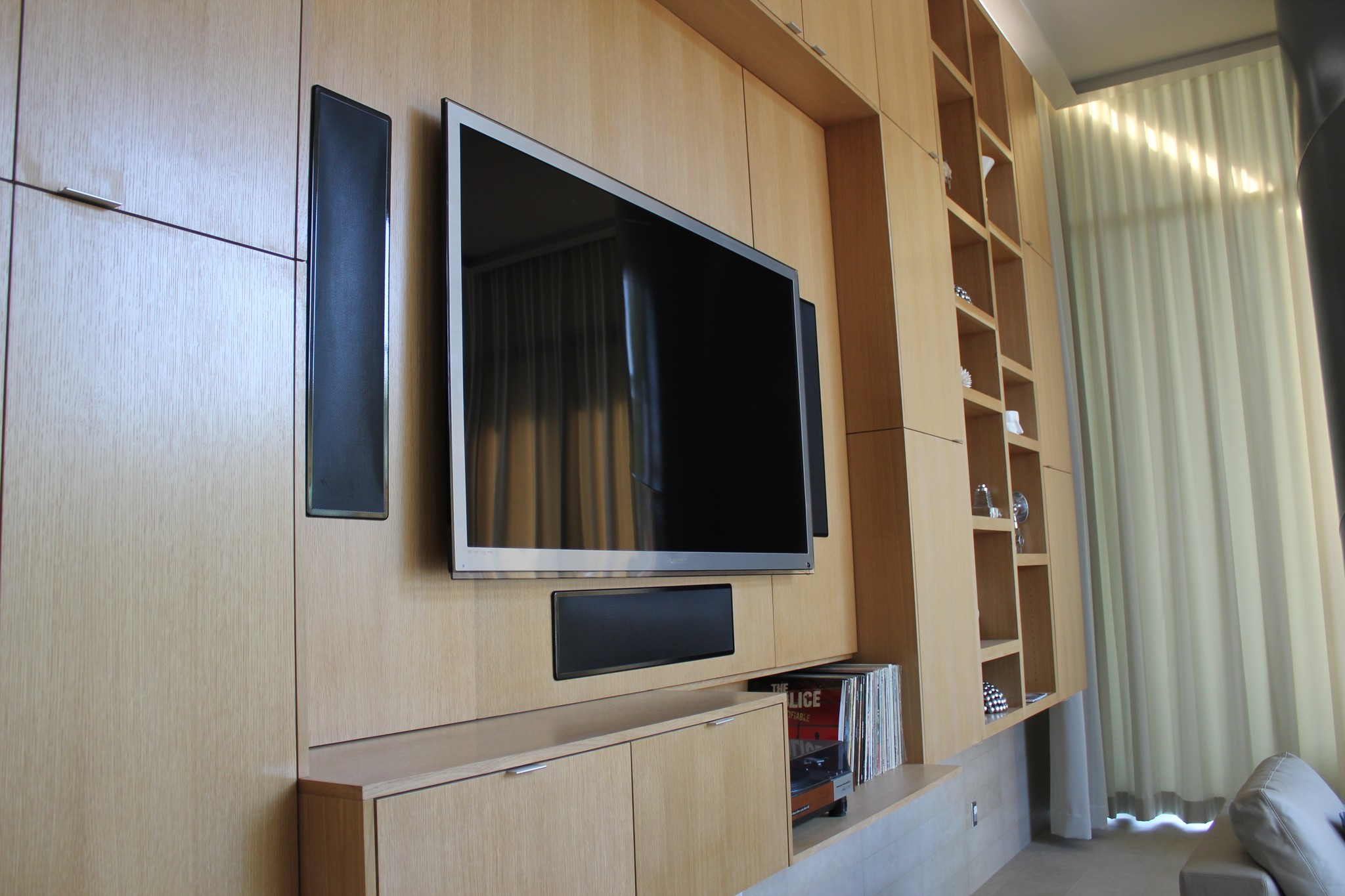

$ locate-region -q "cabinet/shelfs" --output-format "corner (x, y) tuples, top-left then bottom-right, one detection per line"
(921, 0), (1057, 742)
(296, 686), (794, 896)
(652, 0), (880, 129)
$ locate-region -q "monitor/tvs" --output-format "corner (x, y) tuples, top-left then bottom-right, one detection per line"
(435, 96), (816, 579)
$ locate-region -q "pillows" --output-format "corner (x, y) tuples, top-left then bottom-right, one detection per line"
(1229, 751), (1345, 896)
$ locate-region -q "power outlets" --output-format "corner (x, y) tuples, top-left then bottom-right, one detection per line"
(970, 800), (978, 828)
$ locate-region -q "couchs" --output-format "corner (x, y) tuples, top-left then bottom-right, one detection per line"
(1177, 797), (1285, 896)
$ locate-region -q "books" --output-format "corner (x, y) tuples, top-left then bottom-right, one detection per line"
(747, 662), (908, 789)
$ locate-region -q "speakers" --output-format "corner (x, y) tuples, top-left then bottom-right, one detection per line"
(305, 83), (392, 522)
(800, 296), (830, 537)
(550, 583), (735, 681)
